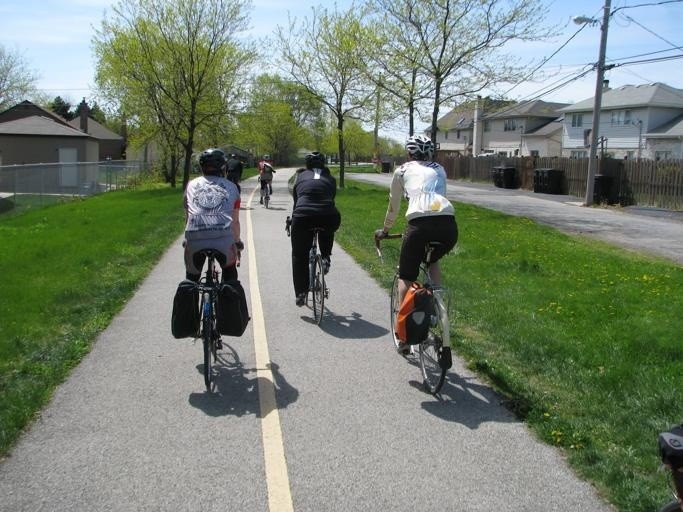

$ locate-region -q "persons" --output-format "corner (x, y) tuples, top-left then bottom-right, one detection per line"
(374, 134), (457, 355)
(225, 153), (242, 186)
(181, 148), (244, 285)
(257, 154), (273, 205)
(287, 151), (342, 306)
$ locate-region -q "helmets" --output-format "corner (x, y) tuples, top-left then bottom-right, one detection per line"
(198, 148), (230, 176)
(263, 154), (270, 160)
(406, 133), (435, 161)
(304, 152), (325, 170)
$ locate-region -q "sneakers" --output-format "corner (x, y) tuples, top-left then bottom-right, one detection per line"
(296, 294), (306, 306)
(269, 188), (272, 194)
(397, 341), (411, 355)
(323, 259), (330, 274)
(260, 200), (263, 204)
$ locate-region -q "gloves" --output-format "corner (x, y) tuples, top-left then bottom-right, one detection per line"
(375, 229), (387, 242)
(235, 240), (244, 250)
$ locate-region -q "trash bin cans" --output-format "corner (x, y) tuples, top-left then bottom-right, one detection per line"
(595, 174), (619, 204)
(382, 163), (389, 173)
(492, 167), (518, 188)
(533, 168), (566, 194)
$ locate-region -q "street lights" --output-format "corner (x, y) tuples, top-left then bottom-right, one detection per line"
(573, 16), (607, 202)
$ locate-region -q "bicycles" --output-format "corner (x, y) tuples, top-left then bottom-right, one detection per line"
(286, 215), (329, 325)
(182, 239), (244, 385)
(375, 233), (452, 393)
(258, 177), (270, 207)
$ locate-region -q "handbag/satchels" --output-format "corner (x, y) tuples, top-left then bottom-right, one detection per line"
(395, 281), (433, 346)
(171, 279), (200, 339)
(217, 280), (249, 336)
(262, 163), (273, 174)
